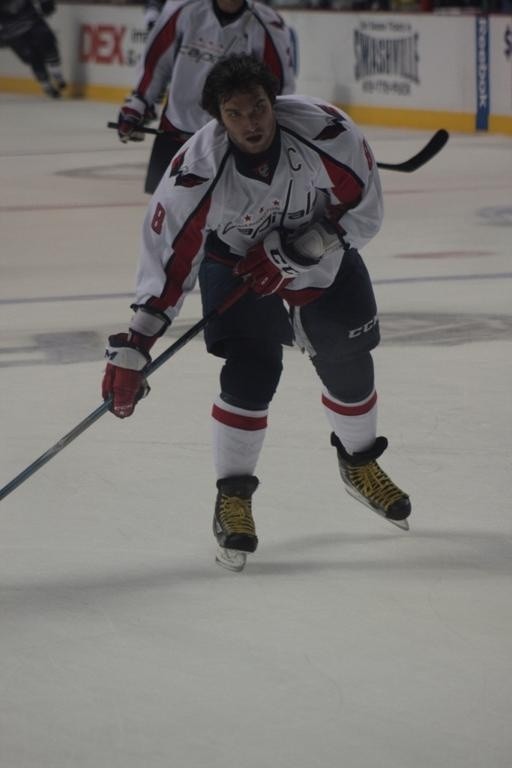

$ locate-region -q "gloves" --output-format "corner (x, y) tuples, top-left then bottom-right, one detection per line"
(102, 332), (152, 418)
(118, 89), (157, 143)
(226, 226), (322, 302)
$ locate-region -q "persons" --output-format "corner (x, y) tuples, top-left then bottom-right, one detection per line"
(112, 0), (303, 199)
(1, 0), (68, 97)
(99, 48), (412, 553)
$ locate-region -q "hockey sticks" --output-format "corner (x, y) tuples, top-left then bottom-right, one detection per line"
(107, 122), (448, 173)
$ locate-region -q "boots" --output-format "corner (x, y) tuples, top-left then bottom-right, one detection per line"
(331, 432), (411, 519)
(212, 475), (259, 553)
(46, 74), (66, 98)
